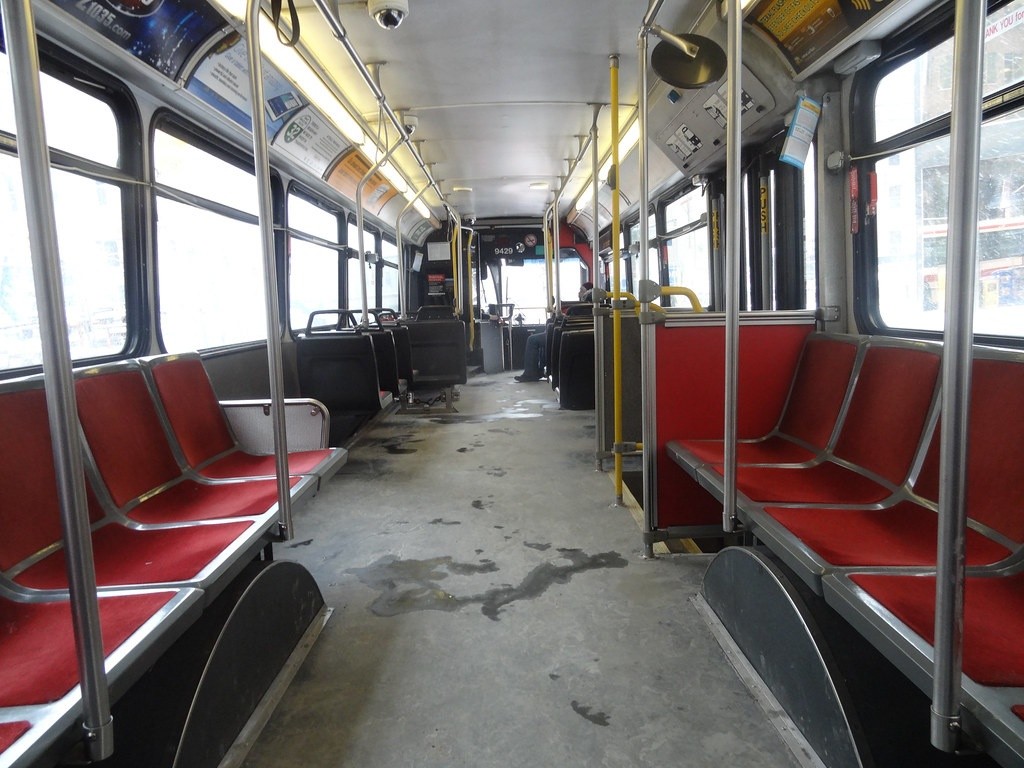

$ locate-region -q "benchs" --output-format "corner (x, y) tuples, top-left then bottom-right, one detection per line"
(378, 307), (468, 410)
(0, 352), (348, 768)
(542, 301), (612, 410)
(666, 333), (1024, 768)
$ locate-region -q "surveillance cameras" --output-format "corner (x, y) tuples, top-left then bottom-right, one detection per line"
(464, 214), (477, 225)
(368, 0), (409, 31)
(403, 116), (417, 134)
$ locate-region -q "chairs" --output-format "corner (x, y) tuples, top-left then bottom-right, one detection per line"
(296, 306), (418, 410)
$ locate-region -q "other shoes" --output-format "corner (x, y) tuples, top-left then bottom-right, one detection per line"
(514, 374), (539, 382)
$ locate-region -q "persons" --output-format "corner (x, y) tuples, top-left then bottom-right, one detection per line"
(514, 282), (593, 382)
